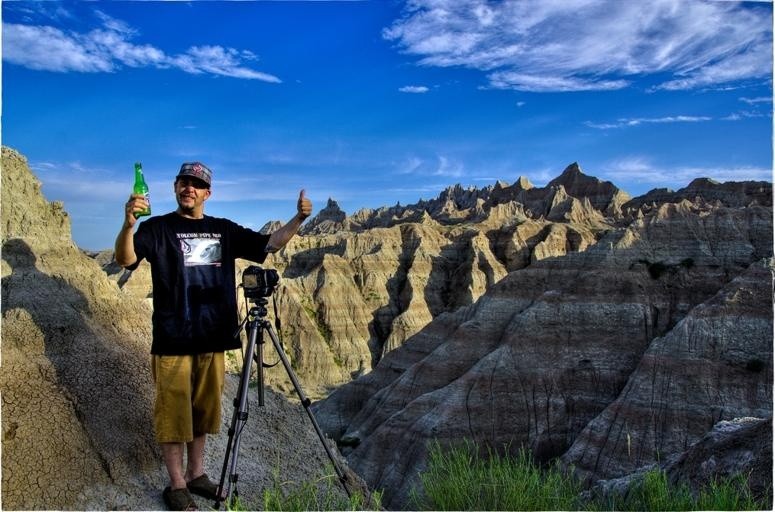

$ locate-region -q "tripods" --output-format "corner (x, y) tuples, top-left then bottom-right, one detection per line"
(214, 297), (352, 512)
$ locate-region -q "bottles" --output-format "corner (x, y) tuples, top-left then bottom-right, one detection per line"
(132, 162), (152, 217)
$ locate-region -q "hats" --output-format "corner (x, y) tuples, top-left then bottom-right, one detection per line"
(176, 163), (212, 187)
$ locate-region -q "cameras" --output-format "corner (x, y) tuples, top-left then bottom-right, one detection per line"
(242, 266), (279, 297)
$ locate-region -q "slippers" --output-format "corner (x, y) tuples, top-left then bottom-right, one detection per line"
(162, 485), (197, 510)
(186, 473), (227, 501)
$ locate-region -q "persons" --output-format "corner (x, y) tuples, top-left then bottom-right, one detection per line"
(114, 162), (314, 510)
